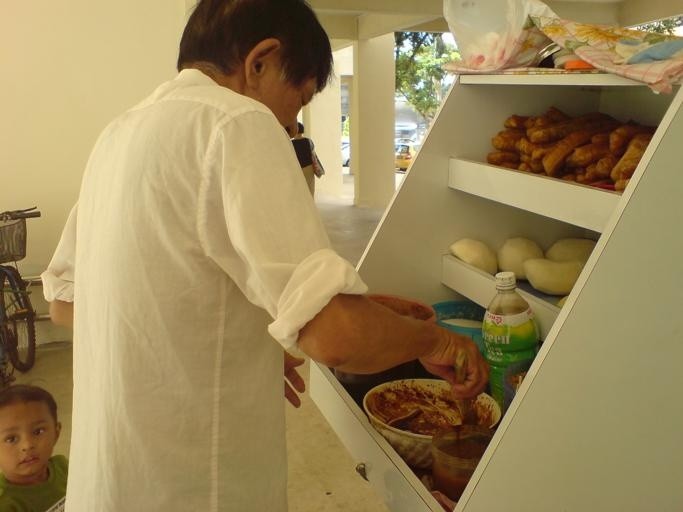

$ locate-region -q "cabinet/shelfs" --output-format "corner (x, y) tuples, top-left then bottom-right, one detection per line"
(307, 72), (682, 511)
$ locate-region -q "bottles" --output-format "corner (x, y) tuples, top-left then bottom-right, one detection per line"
(479, 269), (542, 411)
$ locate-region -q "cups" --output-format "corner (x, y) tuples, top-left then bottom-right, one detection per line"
(432, 430), (498, 504)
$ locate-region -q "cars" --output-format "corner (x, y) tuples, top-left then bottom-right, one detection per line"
(340, 120), (424, 174)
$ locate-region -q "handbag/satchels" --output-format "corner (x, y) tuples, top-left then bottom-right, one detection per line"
(308, 138), (326, 179)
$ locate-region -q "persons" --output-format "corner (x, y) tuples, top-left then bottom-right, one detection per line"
(0, 385), (69, 512)
(39, 0), (490, 512)
(291, 122), (315, 200)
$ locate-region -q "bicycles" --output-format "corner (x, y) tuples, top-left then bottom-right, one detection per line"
(1, 204), (47, 393)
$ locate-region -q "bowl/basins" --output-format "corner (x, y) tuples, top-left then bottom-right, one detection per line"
(364, 377), (503, 466)
(428, 297), (486, 356)
